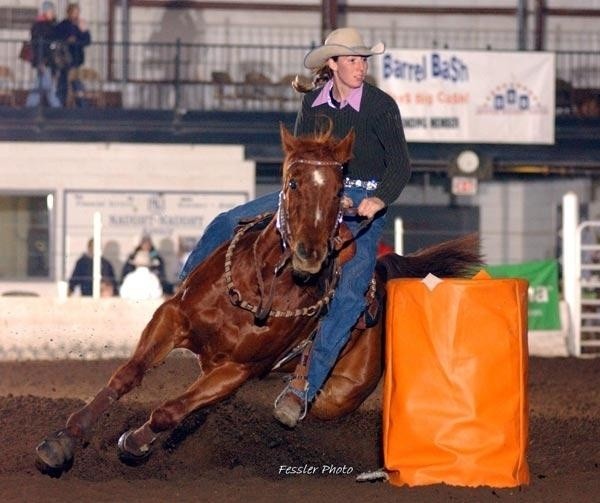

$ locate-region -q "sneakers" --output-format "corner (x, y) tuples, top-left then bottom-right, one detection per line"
(272, 387), (305, 428)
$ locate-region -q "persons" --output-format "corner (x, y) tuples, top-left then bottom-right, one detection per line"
(98, 276), (119, 298)
(53, 4), (92, 108)
(122, 235), (174, 294)
(175, 28), (412, 429)
(24, 0), (65, 108)
(68, 238), (120, 297)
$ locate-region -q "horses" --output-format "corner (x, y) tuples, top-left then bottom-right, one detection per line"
(35, 113), (487, 469)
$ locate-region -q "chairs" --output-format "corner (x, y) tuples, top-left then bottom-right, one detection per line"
(212, 72), (238, 112)
(67, 69), (105, 110)
(0, 67), (16, 107)
(278, 75), (309, 113)
(243, 73), (274, 111)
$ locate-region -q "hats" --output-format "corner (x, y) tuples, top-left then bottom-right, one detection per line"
(303, 27), (386, 69)
